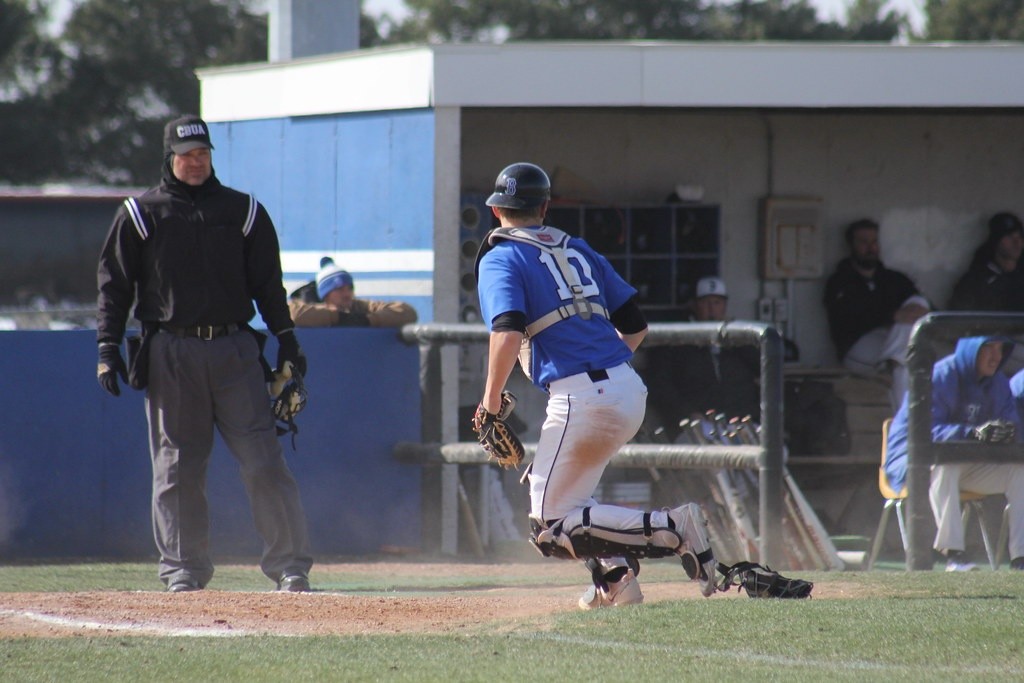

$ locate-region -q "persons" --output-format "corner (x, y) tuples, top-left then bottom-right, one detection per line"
(647, 277), (762, 444)
(952, 212), (1024, 378)
(288, 257), (418, 328)
(885, 334), (1024, 572)
(472, 163), (717, 610)
(822, 219), (932, 415)
(97, 117), (316, 592)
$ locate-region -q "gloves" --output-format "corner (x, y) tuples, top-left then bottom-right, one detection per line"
(1000, 421), (1016, 444)
(275, 330), (307, 380)
(970, 421), (1005, 443)
(97, 343), (128, 397)
(338, 310), (369, 327)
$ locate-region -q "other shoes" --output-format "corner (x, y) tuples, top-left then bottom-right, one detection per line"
(168, 574), (198, 592)
(278, 569), (310, 592)
(1010, 558), (1024, 571)
(946, 554), (978, 572)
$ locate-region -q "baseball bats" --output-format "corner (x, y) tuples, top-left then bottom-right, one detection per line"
(457, 478), (484, 559)
(634, 408), (845, 570)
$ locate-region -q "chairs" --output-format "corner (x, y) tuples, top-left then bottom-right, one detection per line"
(866, 419), (995, 573)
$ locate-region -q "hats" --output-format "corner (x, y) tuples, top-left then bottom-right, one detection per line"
(696, 279), (728, 299)
(990, 213), (1020, 235)
(316, 257), (353, 299)
(165, 115), (215, 155)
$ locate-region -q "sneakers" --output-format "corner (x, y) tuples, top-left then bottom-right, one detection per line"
(579, 569), (644, 606)
(662, 502), (716, 597)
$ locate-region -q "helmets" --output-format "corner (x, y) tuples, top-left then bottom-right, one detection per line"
(486, 162), (550, 210)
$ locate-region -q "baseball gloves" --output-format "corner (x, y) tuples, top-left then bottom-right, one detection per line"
(269, 361), (308, 422)
(474, 391), (526, 465)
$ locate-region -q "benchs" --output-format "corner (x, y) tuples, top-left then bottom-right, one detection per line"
(779, 369), (897, 537)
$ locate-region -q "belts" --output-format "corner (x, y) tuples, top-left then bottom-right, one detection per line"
(587, 369), (608, 382)
(158, 324), (237, 340)
